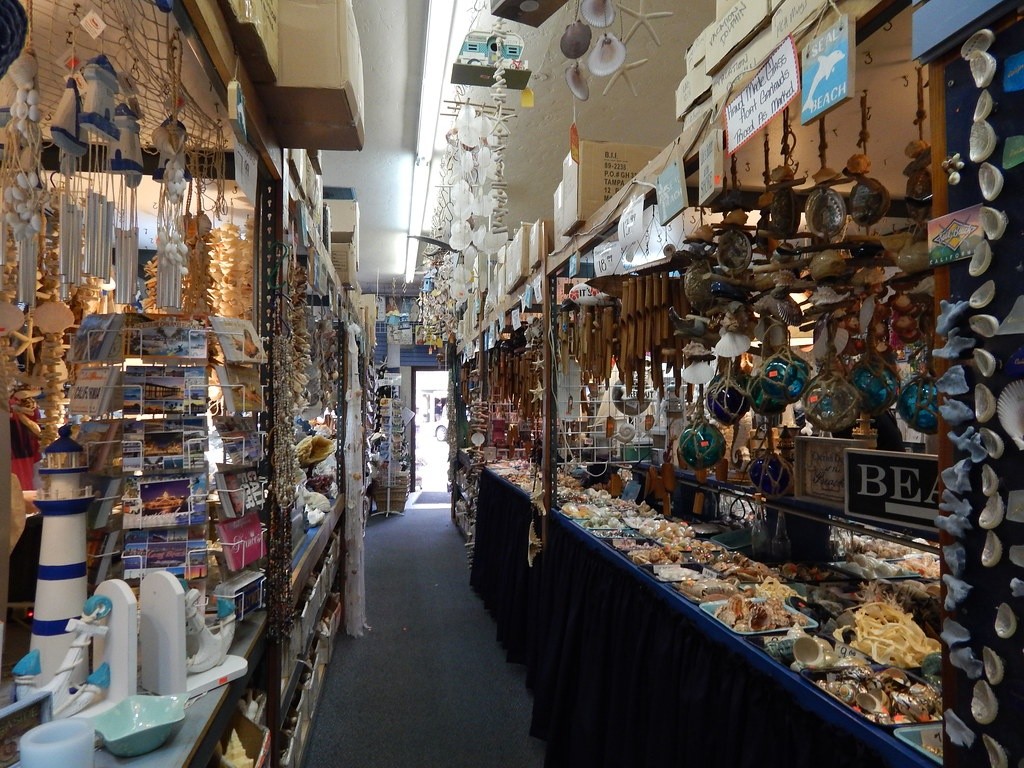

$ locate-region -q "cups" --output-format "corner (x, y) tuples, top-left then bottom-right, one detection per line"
(20, 717), (95, 768)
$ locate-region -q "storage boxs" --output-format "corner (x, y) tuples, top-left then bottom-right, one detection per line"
(220, 0), (377, 341)
(677, 0), (831, 129)
(456, 139), (666, 342)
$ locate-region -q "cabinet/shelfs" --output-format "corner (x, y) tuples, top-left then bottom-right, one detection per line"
(0, 0), (410, 768)
(450, 0), (1024, 768)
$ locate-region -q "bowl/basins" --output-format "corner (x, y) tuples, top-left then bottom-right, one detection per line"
(91, 692), (191, 757)
